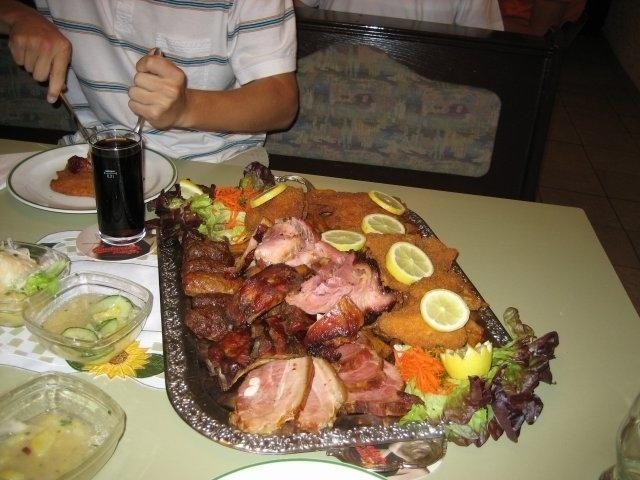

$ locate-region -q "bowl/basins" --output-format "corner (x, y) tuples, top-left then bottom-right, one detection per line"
(0, 238), (71, 328)
(1, 371), (127, 478)
(19, 270), (155, 369)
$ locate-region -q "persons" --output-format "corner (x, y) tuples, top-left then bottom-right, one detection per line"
(499, 2), (561, 37)
(309, 0), (504, 36)
(1, 0), (298, 167)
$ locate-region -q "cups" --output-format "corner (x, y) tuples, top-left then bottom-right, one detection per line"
(88, 129), (147, 247)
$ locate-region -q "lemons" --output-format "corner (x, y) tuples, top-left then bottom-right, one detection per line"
(385, 241), (435, 284)
(321, 229), (366, 251)
(178, 179), (203, 199)
(369, 189), (405, 216)
(249, 183), (287, 209)
(361, 213), (406, 236)
(419, 288), (471, 332)
(440, 339), (493, 377)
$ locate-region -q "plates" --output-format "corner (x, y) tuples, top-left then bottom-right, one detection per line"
(5, 142), (178, 214)
(210, 455), (389, 479)
(77, 223), (156, 262)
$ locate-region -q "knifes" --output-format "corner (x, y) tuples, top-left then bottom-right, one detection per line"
(58, 93), (95, 146)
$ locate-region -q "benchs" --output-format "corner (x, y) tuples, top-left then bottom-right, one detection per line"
(0, 1), (561, 208)
